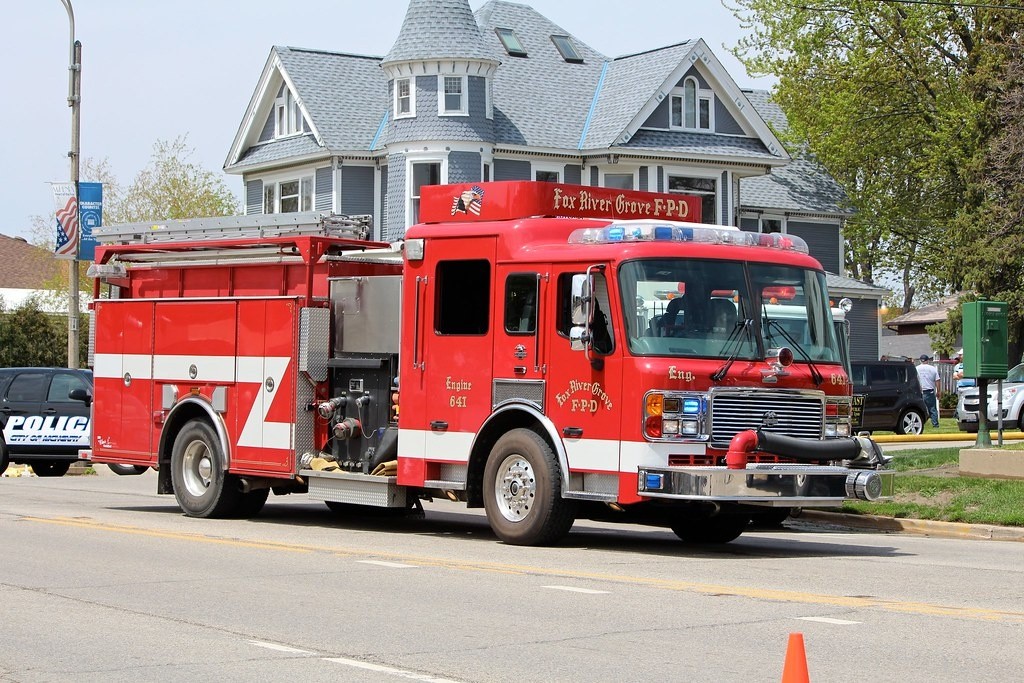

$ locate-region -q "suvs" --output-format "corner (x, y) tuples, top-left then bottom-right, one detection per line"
(850, 357), (931, 440)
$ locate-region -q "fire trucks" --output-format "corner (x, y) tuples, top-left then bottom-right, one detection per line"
(79, 179), (896, 544)
(638, 281), (854, 418)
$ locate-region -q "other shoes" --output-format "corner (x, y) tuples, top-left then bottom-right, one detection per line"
(933, 425), (940, 428)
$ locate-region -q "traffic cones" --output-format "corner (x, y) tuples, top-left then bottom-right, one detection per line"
(779, 631), (813, 683)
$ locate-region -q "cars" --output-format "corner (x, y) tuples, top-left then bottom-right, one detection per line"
(0, 367), (150, 478)
(955, 362), (1024, 436)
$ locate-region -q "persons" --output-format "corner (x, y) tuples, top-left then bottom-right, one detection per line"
(953, 349), (963, 403)
(915, 354), (940, 427)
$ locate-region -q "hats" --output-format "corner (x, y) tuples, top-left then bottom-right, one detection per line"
(956, 348), (964, 355)
(919, 354), (932, 361)
